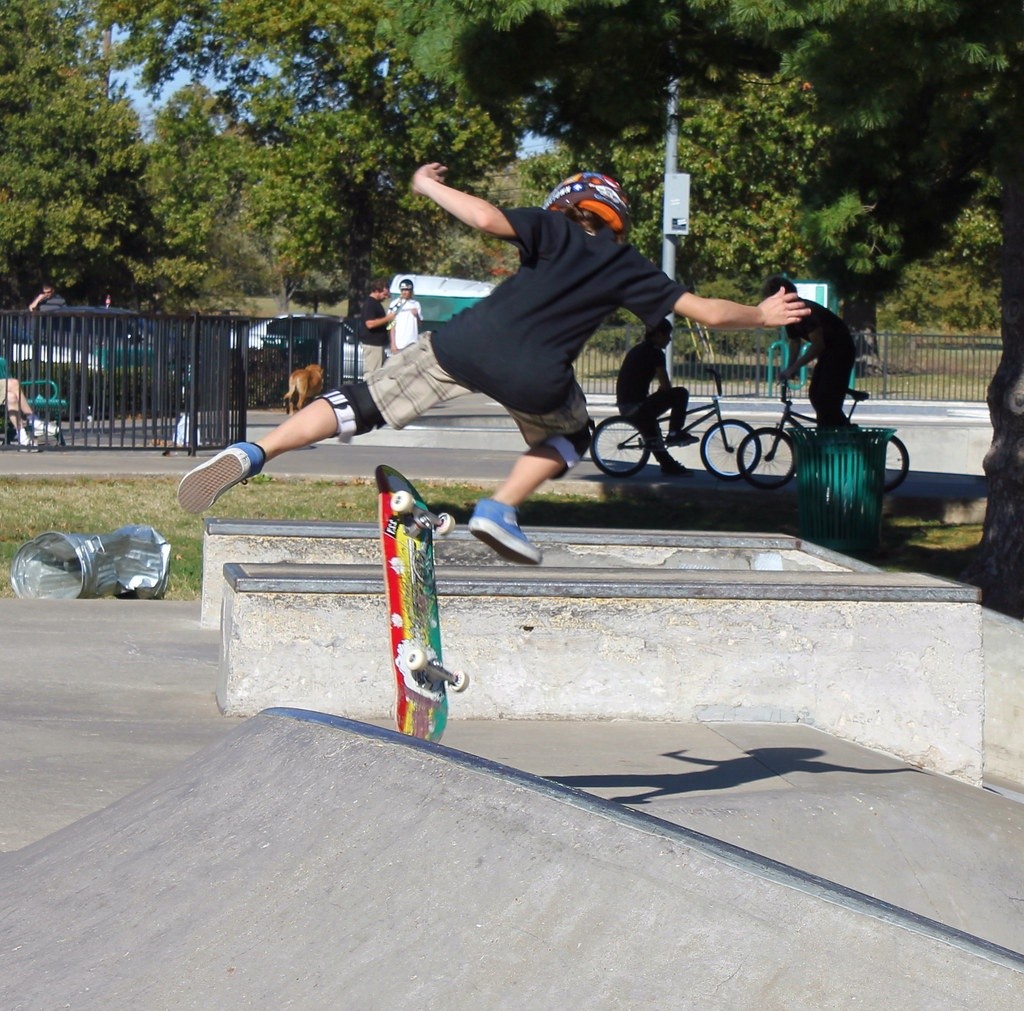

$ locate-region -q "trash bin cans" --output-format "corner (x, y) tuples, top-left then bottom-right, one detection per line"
(783, 426), (899, 568)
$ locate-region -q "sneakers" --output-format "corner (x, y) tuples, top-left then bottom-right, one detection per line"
(469, 499), (543, 566)
(177, 442), (263, 514)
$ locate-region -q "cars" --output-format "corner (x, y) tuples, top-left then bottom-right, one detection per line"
(0, 305), (396, 390)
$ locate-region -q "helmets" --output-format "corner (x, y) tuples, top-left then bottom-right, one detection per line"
(543, 171), (633, 246)
(399, 279), (413, 290)
(645, 318), (672, 336)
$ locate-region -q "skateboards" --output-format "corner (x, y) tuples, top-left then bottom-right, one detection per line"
(370, 462), (471, 749)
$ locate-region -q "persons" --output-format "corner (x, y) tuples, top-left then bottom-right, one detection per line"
(0, 356), (61, 453)
(386, 279), (424, 354)
(28, 282), (69, 312)
(175, 159), (812, 567)
(616, 319), (701, 477)
(359, 277), (397, 383)
(757, 276), (858, 430)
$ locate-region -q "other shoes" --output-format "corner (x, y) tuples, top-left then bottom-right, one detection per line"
(665, 431), (701, 446)
(660, 461), (695, 477)
(32, 422), (59, 436)
(17, 440), (40, 453)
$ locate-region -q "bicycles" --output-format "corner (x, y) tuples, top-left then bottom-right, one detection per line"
(736, 376), (911, 494)
(589, 367), (762, 482)
